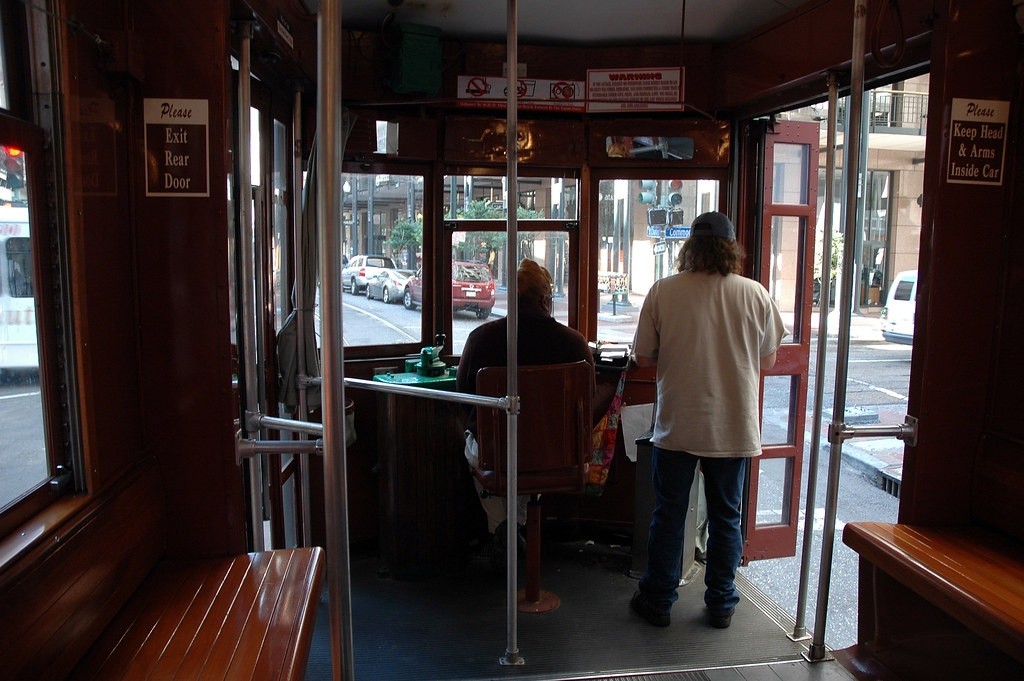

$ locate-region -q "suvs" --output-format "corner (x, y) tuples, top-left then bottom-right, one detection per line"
(341, 254), (398, 295)
(403, 258), (495, 320)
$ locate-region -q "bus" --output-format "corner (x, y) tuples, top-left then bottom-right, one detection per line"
(0, 207), (41, 372)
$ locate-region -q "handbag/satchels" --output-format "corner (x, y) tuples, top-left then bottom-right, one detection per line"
(584, 369), (626, 497)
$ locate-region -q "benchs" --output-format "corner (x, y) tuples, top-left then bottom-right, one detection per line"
(842, 519), (1024, 681)
(62, 544), (326, 681)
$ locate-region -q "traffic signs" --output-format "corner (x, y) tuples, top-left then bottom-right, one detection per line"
(652, 241), (667, 255)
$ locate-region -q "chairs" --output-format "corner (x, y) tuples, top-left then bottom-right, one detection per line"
(463, 359), (595, 613)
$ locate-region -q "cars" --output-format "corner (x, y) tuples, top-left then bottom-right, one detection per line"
(341, 254), (350, 272)
(366, 270), (419, 304)
(879, 270), (919, 345)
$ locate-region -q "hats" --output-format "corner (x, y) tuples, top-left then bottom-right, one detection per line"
(690, 211), (735, 239)
(518, 259), (552, 296)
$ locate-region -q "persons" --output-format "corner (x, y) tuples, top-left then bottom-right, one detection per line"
(456, 258), (597, 568)
(631, 211), (785, 631)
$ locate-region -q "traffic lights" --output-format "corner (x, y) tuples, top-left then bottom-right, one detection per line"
(648, 209), (666, 225)
(637, 179), (661, 209)
(3, 144), (27, 190)
(662, 180), (684, 208)
(669, 210), (684, 227)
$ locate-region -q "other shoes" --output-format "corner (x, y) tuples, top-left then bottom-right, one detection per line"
(631, 590), (671, 628)
(707, 606), (735, 629)
(495, 524), (528, 567)
